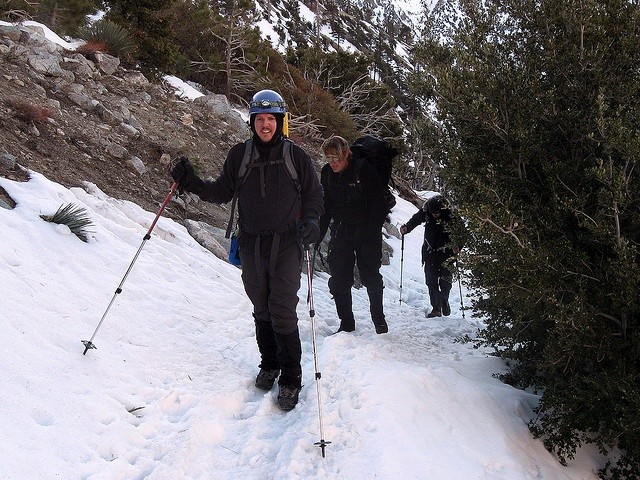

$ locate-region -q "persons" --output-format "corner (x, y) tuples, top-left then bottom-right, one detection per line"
(400, 196), (467, 317)
(313, 133), (396, 334)
(169, 90), (325, 410)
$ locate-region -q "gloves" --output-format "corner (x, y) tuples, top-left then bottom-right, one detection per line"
(295, 216), (320, 245)
(170, 156), (201, 193)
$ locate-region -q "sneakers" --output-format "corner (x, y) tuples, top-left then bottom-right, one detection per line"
(278, 372), (301, 411)
(372, 311), (388, 334)
(442, 300), (451, 316)
(255, 365), (280, 390)
(428, 309), (442, 317)
(332, 319), (355, 335)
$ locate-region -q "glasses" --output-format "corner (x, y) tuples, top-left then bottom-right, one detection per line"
(430, 211), (440, 214)
(325, 154), (344, 162)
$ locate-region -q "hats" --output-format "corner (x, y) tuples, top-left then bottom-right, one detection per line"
(428, 196), (444, 213)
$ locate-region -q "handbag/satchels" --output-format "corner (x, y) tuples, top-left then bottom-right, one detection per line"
(228, 234), (241, 265)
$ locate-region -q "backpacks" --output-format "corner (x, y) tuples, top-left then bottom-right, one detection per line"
(323, 135), (392, 197)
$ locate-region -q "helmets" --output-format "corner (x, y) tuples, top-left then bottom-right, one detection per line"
(249, 89), (286, 128)
